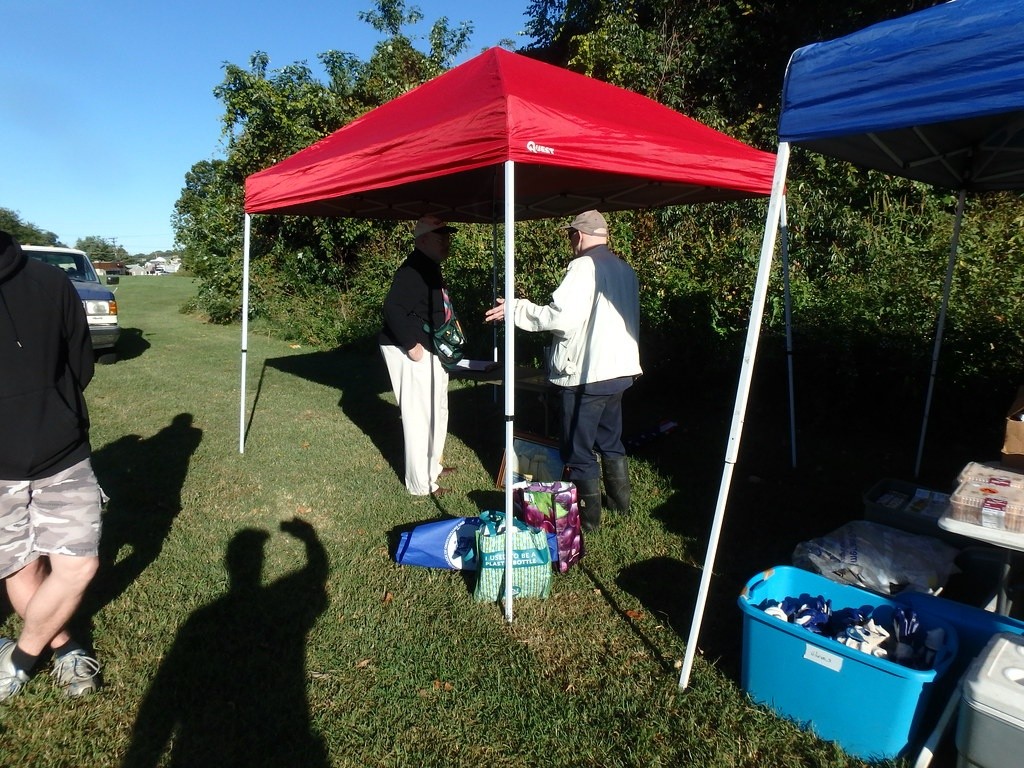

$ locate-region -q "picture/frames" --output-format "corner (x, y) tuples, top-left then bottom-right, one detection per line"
(496, 429), (568, 490)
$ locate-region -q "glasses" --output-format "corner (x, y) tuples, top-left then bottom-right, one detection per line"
(424, 234), (452, 245)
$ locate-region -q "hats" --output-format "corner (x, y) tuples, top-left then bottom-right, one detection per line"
(415, 216), (459, 241)
(560, 209), (608, 238)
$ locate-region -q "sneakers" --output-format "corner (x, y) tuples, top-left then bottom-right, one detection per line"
(0, 638), (30, 703)
(50, 648), (97, 697)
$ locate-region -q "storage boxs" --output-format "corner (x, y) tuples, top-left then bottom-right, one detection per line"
(955, 631), (1024, 768)
(1001, 386), (1024, 467)
(738, 565), (956, 765)
(864, 478), (996, 550)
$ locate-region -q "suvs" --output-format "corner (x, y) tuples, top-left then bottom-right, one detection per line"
(15, 244), (122, 365)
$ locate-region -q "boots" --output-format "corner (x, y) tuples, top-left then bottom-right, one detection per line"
(573, 478), (601, 532)
(602, 457), (630, 514)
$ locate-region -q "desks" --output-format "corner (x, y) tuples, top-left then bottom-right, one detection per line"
(937, 462), (1024, 618)
(451, 366), (557, 439)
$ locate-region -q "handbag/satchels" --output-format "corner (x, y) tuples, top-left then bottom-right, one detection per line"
(473, 511), (552, 601)
(432, 316), (468, 370)
(523, 479), (585, 574)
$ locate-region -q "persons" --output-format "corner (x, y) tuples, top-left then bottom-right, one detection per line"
(486, 208), (643, 526)
(376, 215), (458, 500)
(0, 228), (111, 702)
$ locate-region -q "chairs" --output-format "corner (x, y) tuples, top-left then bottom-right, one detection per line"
(68, 271), (86, 280)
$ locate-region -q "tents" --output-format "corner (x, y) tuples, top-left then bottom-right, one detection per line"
(666, 0), (1024, 690)
(240, 46), (795, 626)
